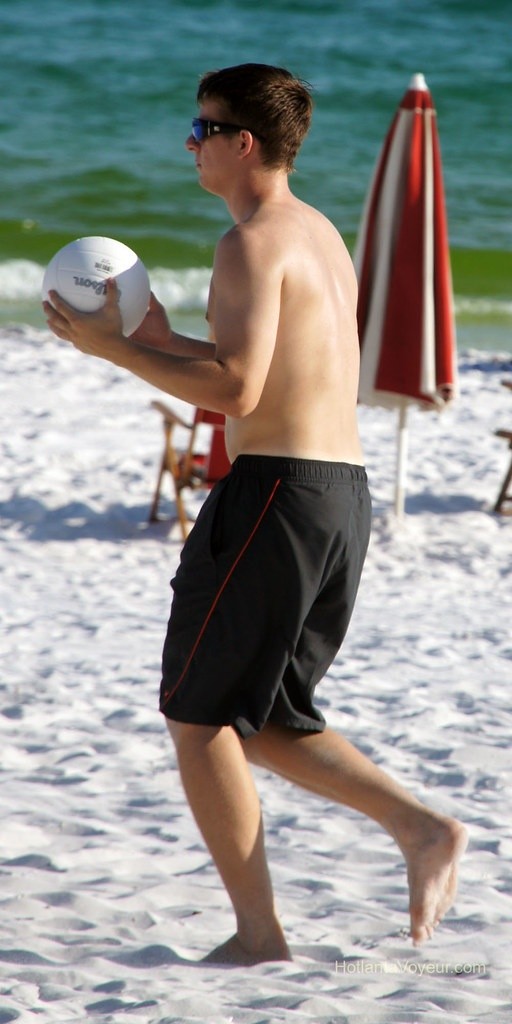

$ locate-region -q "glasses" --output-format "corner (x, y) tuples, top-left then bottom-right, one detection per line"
(189, 117), (254, 143)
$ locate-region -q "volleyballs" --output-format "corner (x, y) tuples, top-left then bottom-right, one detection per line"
(41, 236), (151, 337)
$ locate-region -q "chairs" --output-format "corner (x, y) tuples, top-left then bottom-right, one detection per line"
(145, 402), (232, 545)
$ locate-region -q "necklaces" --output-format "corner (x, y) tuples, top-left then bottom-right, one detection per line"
(42, 62), (467, 973)
